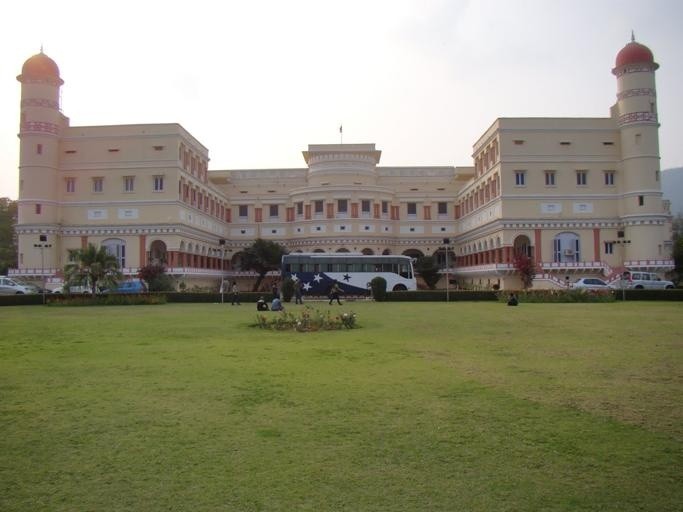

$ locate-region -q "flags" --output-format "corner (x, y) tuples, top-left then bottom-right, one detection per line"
(339, 124), (342, 134)
(622, 271), (632, 282)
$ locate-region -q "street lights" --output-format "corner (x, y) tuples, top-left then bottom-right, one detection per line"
(217, 238), (226, 304)
(441, 236), (451, 301)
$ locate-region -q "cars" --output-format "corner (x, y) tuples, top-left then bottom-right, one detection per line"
(99, 277), (149, 293)
(0, 275), (38, 295)
(25, 282), (51, 293)
(571, 277), (617, 288)
(51, 279), (90, 294)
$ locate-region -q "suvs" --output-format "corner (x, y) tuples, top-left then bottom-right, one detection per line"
(620, 270), (676, 290)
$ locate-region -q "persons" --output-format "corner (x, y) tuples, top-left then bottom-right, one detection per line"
(294, 284), (302, 304)
(327, 278), (344, 305)
(271, 285), (277, 295)
(254, 295), (269, 311)
(270, 296), (284, 311)
(506, 292), (518, 306)
(230, 281), (241, 305)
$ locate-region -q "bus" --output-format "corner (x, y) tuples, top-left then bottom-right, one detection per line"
(280, 252), (418, 300)
